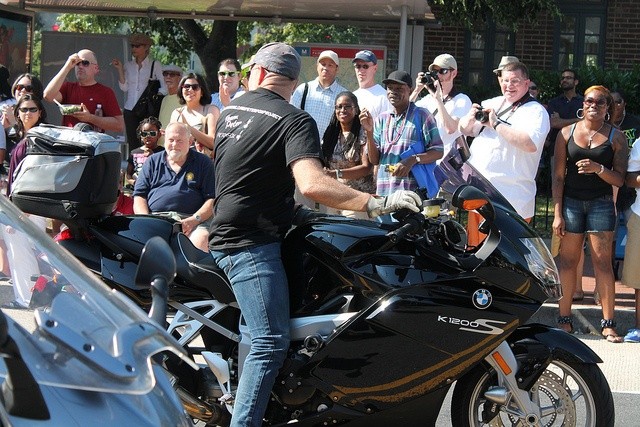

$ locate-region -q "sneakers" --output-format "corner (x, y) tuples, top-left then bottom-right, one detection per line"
(624, 328), (640, 343)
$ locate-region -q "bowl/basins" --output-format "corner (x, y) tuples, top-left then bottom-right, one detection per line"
(56, 102), (83, 116)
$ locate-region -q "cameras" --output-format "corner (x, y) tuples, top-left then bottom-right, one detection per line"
(474, 106), (497, 123)
(421, 71), (442, 92)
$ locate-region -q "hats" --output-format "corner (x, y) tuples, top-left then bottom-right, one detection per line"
(428, 54), (457, 72)
(493, 55), (520, 73)
(383, 70), (412, 87)
(241, 42), (302, 80)
(352, 50), (377, 64)
(160, 65), (182, 72)
(317, 50), (339, 66)
(128, 33), (152, 45)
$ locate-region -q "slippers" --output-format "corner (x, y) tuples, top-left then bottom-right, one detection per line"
(593, 293), (602, 306)
(555, 295), (584, 303)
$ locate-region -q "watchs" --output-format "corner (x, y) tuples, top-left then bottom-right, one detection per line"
(335, 169), (343, 180)
(595, 164), (605, 175)
(193, 213), (203, 224)
(413, 154), (421, 164)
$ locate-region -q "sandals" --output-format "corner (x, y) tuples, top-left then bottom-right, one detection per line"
(601, 318), (623, 343)
(557, 316), (575, 334)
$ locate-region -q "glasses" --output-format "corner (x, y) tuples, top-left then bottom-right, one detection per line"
(18, 108), (37, 112)
(585, 100), (606, 107)
(335, 105), (355, 110)
(182, 84), (200, 90)
(495, 70), (501, 77)
(432, 68), (453, 75)
(354, 65), (369, 69)
(218, 71), (241, 77)
(140, 129), (157, 137)
(560, 76), (576, 80)
(529, 86), (537, 90)
(131, 43), (144, 48)
(614, 99), (622, 103)
(15, 85), (33, 92)
(246, 66), (270, 79)
(162, 71), (177, 77)
(76, 60), (89, 67)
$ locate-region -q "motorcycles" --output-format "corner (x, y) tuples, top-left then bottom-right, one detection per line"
(1, 193), (198, 423)
(9, 122), (617, 423)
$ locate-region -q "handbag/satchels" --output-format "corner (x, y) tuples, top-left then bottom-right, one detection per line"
(9, 123), (122, 205)
(136, 80), (165, 118)
(195, 104), (215, 160)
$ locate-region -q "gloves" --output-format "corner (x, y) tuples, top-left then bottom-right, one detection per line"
(366, 190), (423, 218)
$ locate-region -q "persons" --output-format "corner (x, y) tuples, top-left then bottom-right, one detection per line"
(351, 50), (394, 121)
(209, 58), (250, 113)
(207, 40), (423, 427)
(320, 91), (377, 196)
(526, 78), (553, 117)
(157, 65), (184, 146)
(112, 33), (169, 152)
(459, 62), (550, 225)
(607, 89), (640, 153)
(0, 93), (49, 310)
(493, 56), (519, 87)
(408, 53), (473, 165)
(43, 48), (125, 133)
(125, 116), (165, 186)
(547, 70), (585, 176)
(289, 50), (348, 149)
(0, 65), (48, 281)
(557, 183), (619, 306)
(170, 72), (220, 159)
(552, 84), (629, 342)
(624, 136), (640, 343)
(133, 122), (215, 253)
(358, 70), (444, 223)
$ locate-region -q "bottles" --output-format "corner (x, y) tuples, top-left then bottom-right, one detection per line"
(93, 102), (105, 133)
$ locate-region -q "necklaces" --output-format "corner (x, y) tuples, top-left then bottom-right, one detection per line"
(611, 117), (625, 129)
(583, 121), (604, 146)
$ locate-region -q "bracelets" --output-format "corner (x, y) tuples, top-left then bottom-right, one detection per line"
(492, 121), (501, 130)
